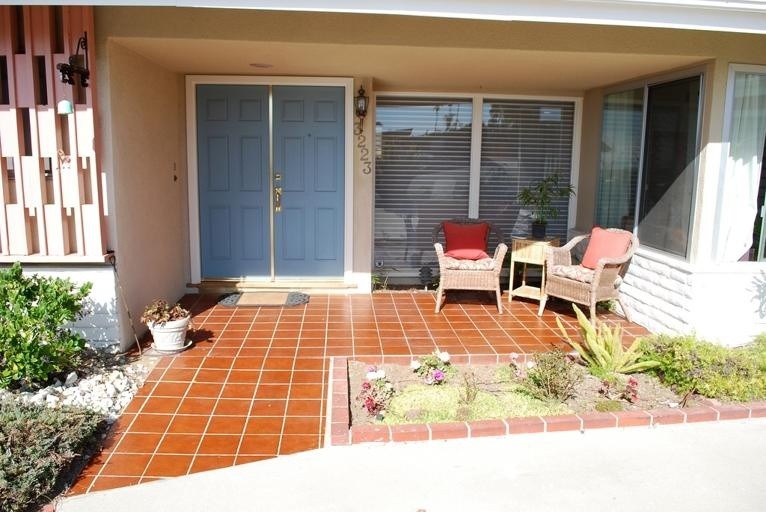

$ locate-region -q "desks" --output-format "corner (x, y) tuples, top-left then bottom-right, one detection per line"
(508, 236), (561, 304)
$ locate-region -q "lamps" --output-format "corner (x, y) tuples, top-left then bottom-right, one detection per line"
(354, 85), (369, 133)
(57, 31), (89, 116)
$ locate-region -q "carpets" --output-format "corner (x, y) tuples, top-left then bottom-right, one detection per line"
(216, 292), (310, 308)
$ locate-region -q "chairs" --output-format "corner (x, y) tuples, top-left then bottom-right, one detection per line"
(432, 218), (509, 314)
(538, 228), (639, 323)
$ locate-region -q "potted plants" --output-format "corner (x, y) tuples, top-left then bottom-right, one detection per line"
(515, 175), (575, 241)
(141, 299), (193, 354)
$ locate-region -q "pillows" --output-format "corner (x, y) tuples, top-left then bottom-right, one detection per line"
(442, 221), (490, 259)
(580, 227), (632, 270)
(552, 266), (622, 288)
(445, 257), (495, 270)
